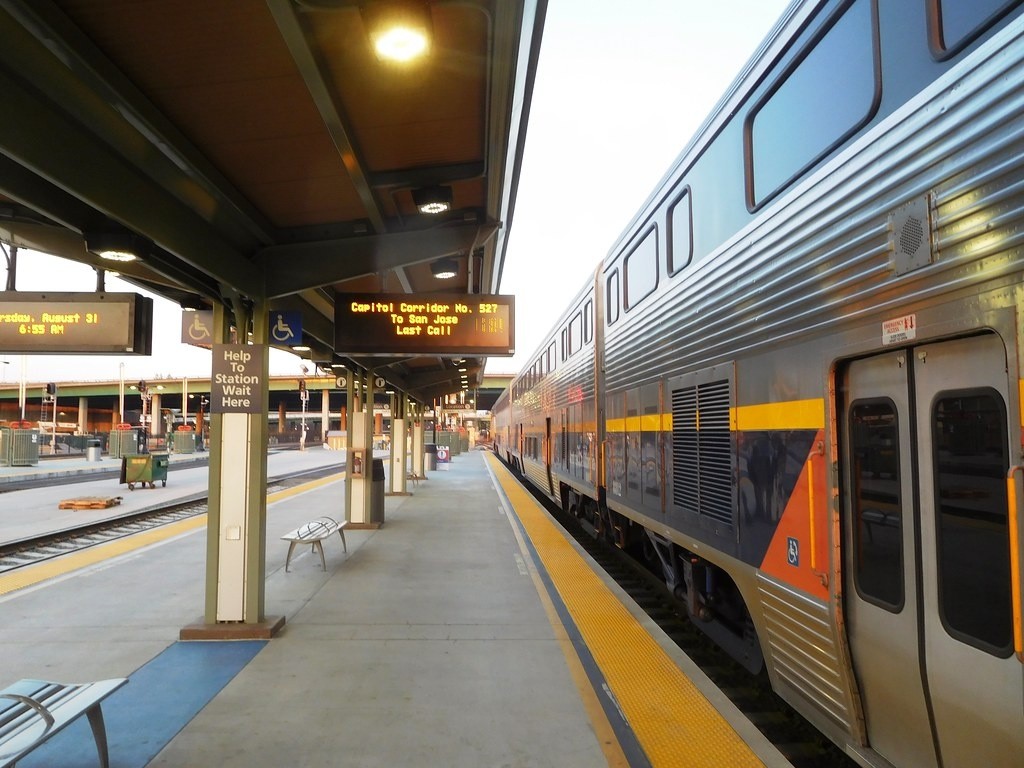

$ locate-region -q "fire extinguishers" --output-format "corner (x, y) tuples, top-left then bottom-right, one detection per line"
(354, 456), (361, 472)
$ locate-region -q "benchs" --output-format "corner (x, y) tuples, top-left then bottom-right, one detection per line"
(0, 678), (130, 768)
(281, 516), (348, 573)
(406, 471), (419, 484)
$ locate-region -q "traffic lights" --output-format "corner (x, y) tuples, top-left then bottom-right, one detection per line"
(47, 383), (55, 394)
(135, 380), (146, 392)
(299, 380), (306, 392)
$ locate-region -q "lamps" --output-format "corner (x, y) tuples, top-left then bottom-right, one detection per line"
(411, 183), (453, 215)
(359, 0), (433, 61)
(430, 260), (458, 280)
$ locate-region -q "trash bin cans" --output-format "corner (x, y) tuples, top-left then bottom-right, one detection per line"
(424, 443), (437, 470)
(371, 457), (385, 524)
(86, 440), (101, 461)
(120, 454), (169, 490)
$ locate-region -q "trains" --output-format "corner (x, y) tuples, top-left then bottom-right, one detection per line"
(490, 0), (1024, 768)
